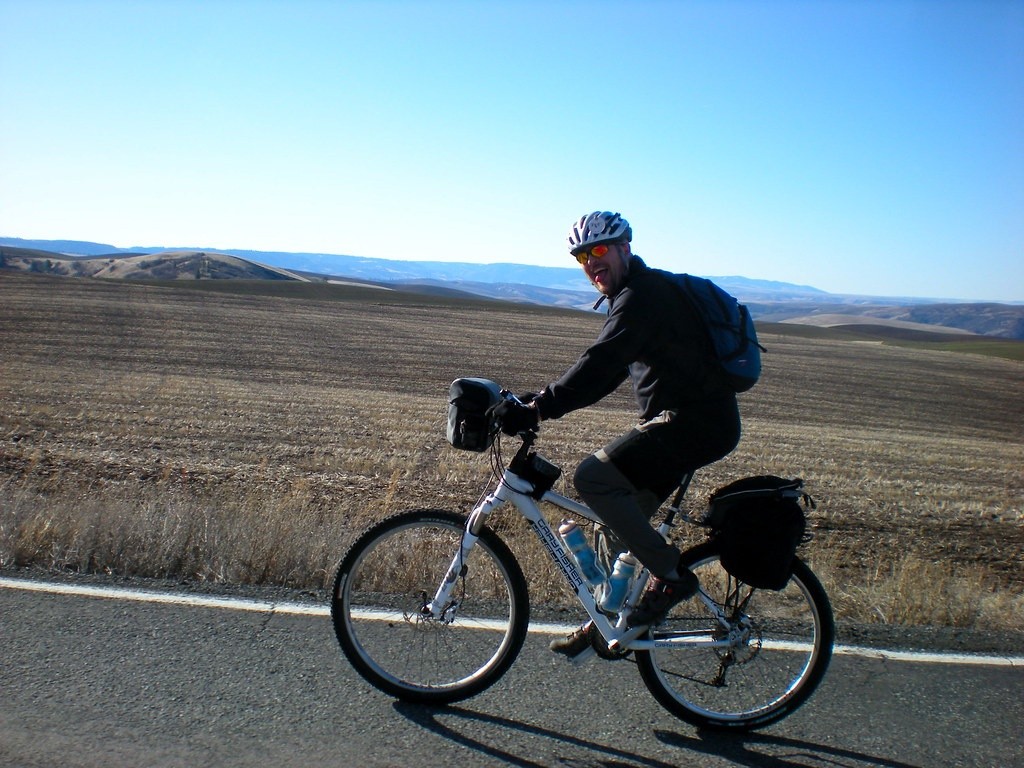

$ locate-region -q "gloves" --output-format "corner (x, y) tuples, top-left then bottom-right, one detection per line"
(513, 393), (537, 404)
(495, 400), (537, 435)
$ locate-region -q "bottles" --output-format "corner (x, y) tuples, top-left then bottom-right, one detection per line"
(559, 517), (636, 613)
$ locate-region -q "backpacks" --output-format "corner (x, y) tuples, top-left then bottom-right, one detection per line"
(622, 268), (767, 393)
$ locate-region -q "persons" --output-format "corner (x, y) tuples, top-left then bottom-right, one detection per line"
(493, 211), (741, 656)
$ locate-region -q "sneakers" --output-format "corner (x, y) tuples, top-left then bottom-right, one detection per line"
(626, 570), (699, 625)
(549, 618), (624, 655)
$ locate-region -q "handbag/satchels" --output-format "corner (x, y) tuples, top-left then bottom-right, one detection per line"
(709, 475), (817, 593)
(447, 378), (503, 452)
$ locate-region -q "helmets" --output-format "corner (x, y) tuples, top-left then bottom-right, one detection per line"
(569, 211), (632, 257)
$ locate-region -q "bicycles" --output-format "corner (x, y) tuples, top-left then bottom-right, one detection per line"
(329, 389), (835, 735)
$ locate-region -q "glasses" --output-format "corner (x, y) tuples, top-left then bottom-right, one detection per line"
(574, 242), (626, 264)
